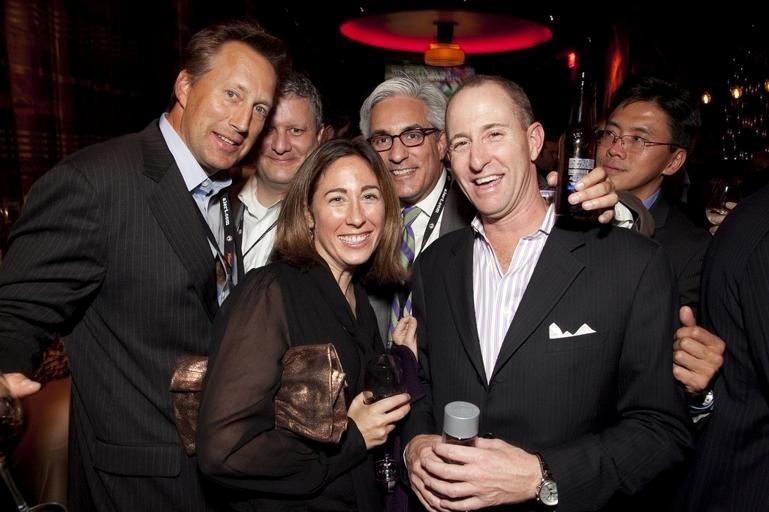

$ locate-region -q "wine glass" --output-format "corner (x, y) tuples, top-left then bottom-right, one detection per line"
(705, 179), (729, 236)
(0, 369), (67, 512)
(363, 363), (406, 485)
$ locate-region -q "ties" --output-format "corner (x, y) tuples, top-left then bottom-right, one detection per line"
(388, 208), (421, 348)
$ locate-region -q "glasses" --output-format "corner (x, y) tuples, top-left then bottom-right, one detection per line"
(366, 127), (438, 152)
(593, 128), (672, 151)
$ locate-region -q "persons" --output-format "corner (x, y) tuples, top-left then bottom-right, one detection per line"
(1, 23), (768, 511)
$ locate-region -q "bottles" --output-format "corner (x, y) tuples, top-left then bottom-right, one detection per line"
(556, 67), (599, 219)
(441, 401), (480, 507)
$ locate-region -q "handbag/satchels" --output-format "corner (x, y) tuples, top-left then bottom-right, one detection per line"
(170, 343), (348, 456)
